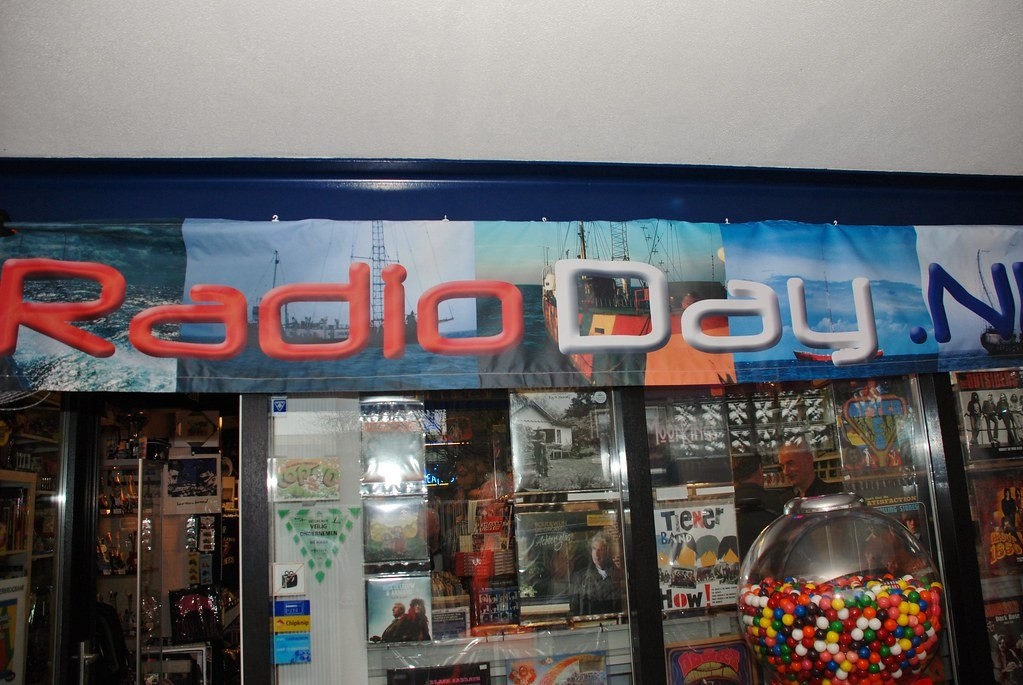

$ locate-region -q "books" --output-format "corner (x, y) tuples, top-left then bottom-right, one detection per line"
(0, 495), (28, 552)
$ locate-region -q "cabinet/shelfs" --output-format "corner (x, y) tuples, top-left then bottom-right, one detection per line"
(0, 406), (241, 684)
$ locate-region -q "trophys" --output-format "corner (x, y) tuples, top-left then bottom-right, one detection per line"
(118, 410), (150, 444)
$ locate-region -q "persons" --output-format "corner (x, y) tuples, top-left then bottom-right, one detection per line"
(479, 591), (518, 625)
(569, 530), (623, 616)
(369, 602), (409, 643)
(996, 392), (1016, 446)
(863, 522), (888, 576)
(1001, 516), (1022, 546)
(719, 453), (784, 582)
(778, 438), (852, 580)
(1016, 507), (1023, 539)
(471, 504), (510, 551)
(881, 515), (913, 579)
(1001, 487), (1016, 528)
(668, 295), (678, 312)
(680, 292), (696, 310)
(290, 315), (339, 330)
(1008, 393), (1023, 441)
(982, 392), (1000, 446)
(1019, 394), (1023, 413)
(990, 635), (1023, 685)
(985, 617), (1013, 652)
(1014, 488), (1023, 513)
(967, 391), (982, 448)
(657, 562), (739, 588)
(406, 310), (415, 324)
(392, 598), (431, 642)
(902, 509), (927, 560)
(530, 425), (551, 478)
(91, 563), (131, 685)
